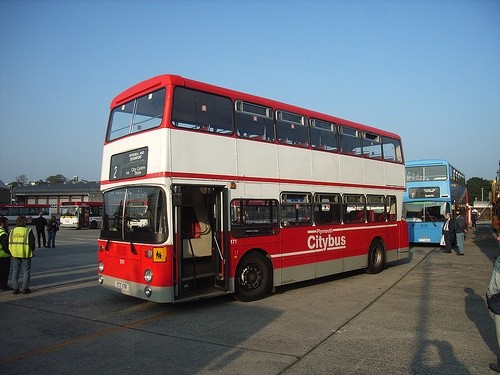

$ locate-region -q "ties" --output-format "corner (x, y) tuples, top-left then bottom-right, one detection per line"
(445, 221), (448, 230)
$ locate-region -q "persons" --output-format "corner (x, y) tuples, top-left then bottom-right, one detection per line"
(36, 211), (49, 248)
(471, 214), (479, 229)
(439, 212), (455, 253)
(454, 210), (468, 256)
(85, 211), (89, 227)
(46, 216), (59, 248)
(3, 216), (36, 295)
(0, 216), (14, 291)
(485, 254), (500, 373)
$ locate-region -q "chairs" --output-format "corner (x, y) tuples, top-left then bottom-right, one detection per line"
(200, 120), (210, 131)
(350, 210), (376, 222)
(385, 155), (394, 161)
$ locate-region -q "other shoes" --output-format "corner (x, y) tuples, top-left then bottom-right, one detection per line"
(22, 288), (32, 295)
(0, 285), (14, 291)
(490, 362), (500, 373)
(12, 288), (21, 295)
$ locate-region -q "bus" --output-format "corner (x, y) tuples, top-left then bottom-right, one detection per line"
(402, 158), (467, 247)
(0, 203), (52, 226)
(97, 74), (410, 304)
(59, 201), (106, 229)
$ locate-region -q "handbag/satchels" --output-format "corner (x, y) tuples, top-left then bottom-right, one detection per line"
(440, 235), (446, 247)
